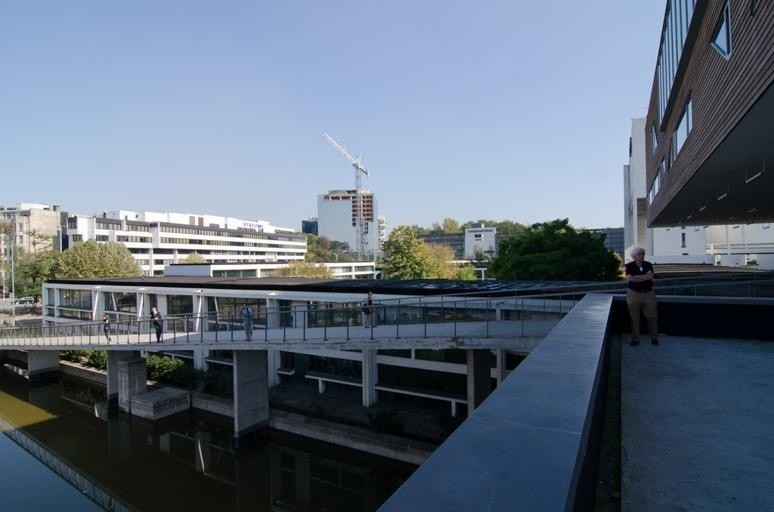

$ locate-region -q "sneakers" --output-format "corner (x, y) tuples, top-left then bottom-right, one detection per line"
(630, 339), (658, 346)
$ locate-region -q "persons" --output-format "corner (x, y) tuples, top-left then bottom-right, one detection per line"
(33, 294), (39, 311)
(150, 307), (163, 343)
(362, 290), (373, 329)
(102, 315), (112, 342)
(241, 302), (254, 341)
(626, 248), (658, 346)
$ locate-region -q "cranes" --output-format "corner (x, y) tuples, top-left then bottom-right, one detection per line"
(320, 132), (370, 260)
